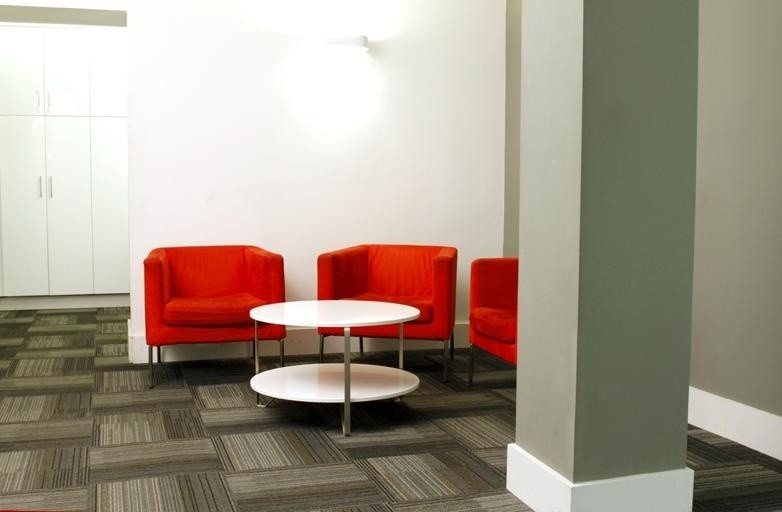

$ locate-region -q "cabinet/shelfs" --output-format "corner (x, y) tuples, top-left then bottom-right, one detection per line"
(0, 21), (127, 297)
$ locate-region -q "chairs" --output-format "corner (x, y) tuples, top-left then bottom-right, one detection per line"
(468, 256), (516, 386)
(317, 244), (458, 383)
(144, 243), (287, 391)
